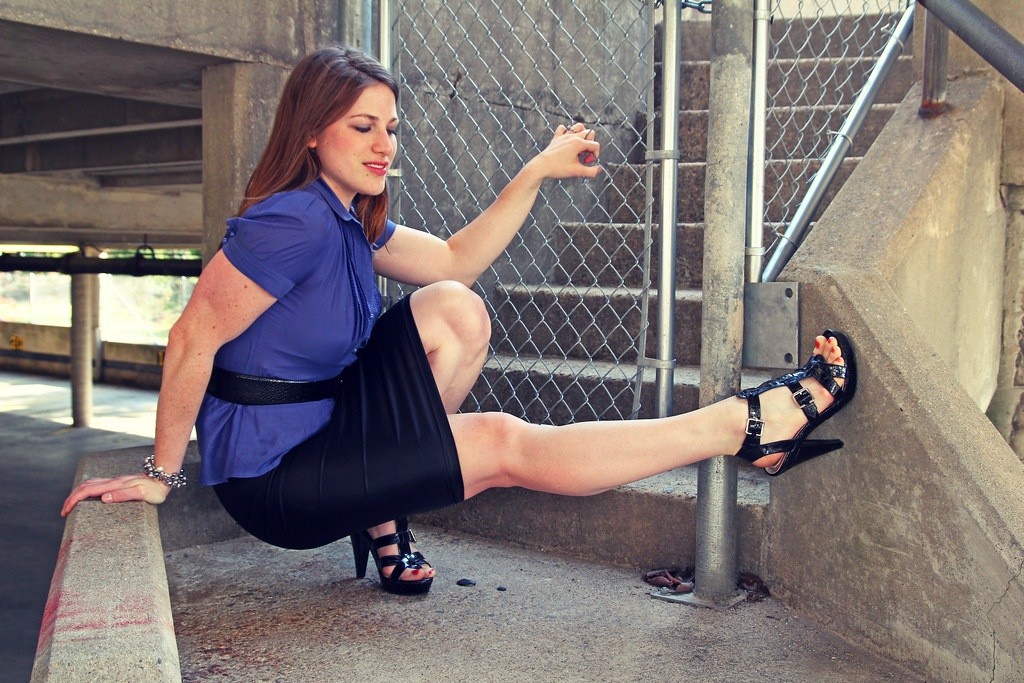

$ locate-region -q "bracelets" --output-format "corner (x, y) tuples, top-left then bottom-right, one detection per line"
(143, 455), (188, 488)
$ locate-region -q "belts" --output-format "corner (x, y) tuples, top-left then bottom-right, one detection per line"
(207, 368), (342, 406)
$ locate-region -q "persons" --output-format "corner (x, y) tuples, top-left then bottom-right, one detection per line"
(59, 45), (858, 596)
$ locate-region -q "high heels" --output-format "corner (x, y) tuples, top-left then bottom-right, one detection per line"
(351, 515), (433, 596)
(735, 329), (857, 477)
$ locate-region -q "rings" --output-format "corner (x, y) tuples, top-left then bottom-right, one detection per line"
(568, 127), (576, 133)
